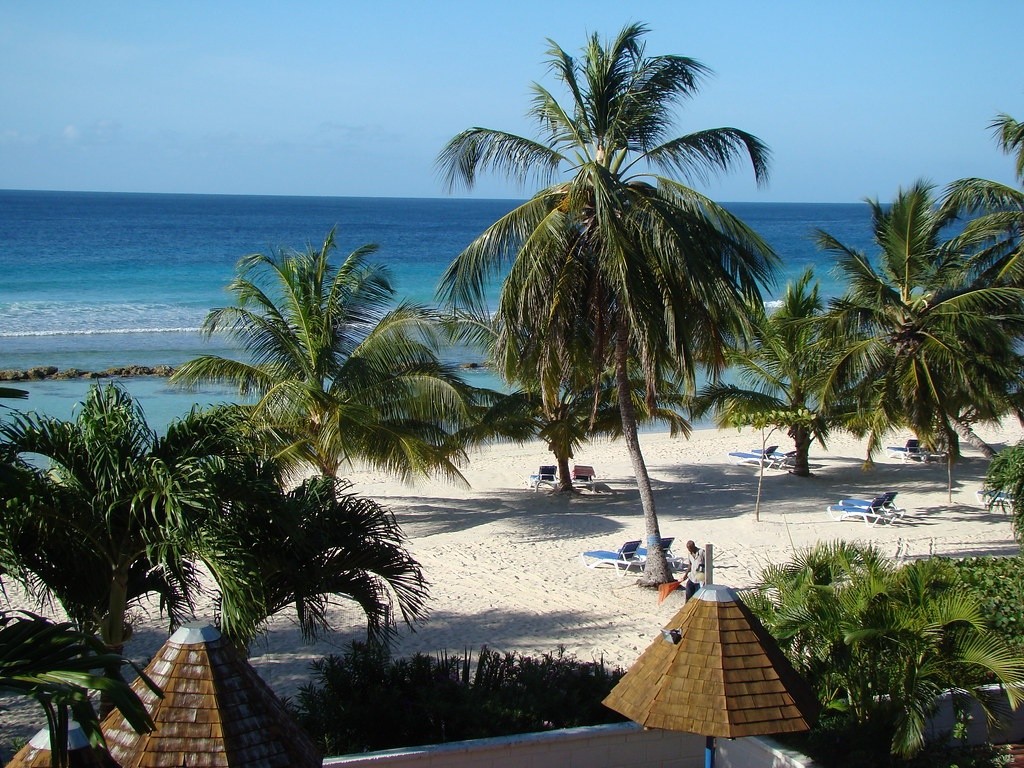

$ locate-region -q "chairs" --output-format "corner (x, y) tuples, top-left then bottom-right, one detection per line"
(618, 536), (685, 571)
(976, 488), (1015, 515)
(752, 450), (799, 468)
(826, 498), (897, 527)
(887, 439), (948, 463)
(570, 465), (596, 492)
(579, 540), (645, 577)
(529, 465), (562, 492)
(839, 491), (907, 521)
(728, 445), (778, 471)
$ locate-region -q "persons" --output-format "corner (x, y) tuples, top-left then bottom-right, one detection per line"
(682, 540), (705, 604)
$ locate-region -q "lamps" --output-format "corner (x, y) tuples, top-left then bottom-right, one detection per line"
(660, 627), (682, 646)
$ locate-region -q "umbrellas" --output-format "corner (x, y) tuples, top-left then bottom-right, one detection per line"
(5, 720), (122, 768)
(90, 622), (324, 768)
(602, 585), (825, 768)
